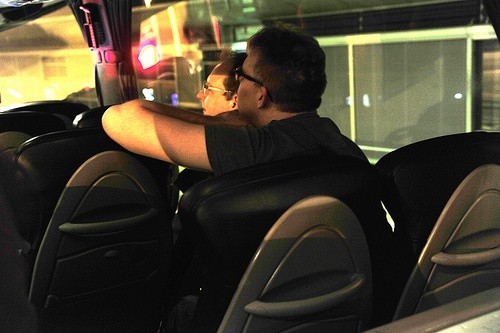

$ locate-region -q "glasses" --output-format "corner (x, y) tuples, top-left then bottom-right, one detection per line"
(203, 80), (234, 98)
(232, 66), (274, 102)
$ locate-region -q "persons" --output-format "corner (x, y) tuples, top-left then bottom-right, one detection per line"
(195, 50), (249, 116)
(102, 22), (372, 176)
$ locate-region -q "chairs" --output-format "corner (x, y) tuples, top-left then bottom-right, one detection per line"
(1, 100), (500, 333)
